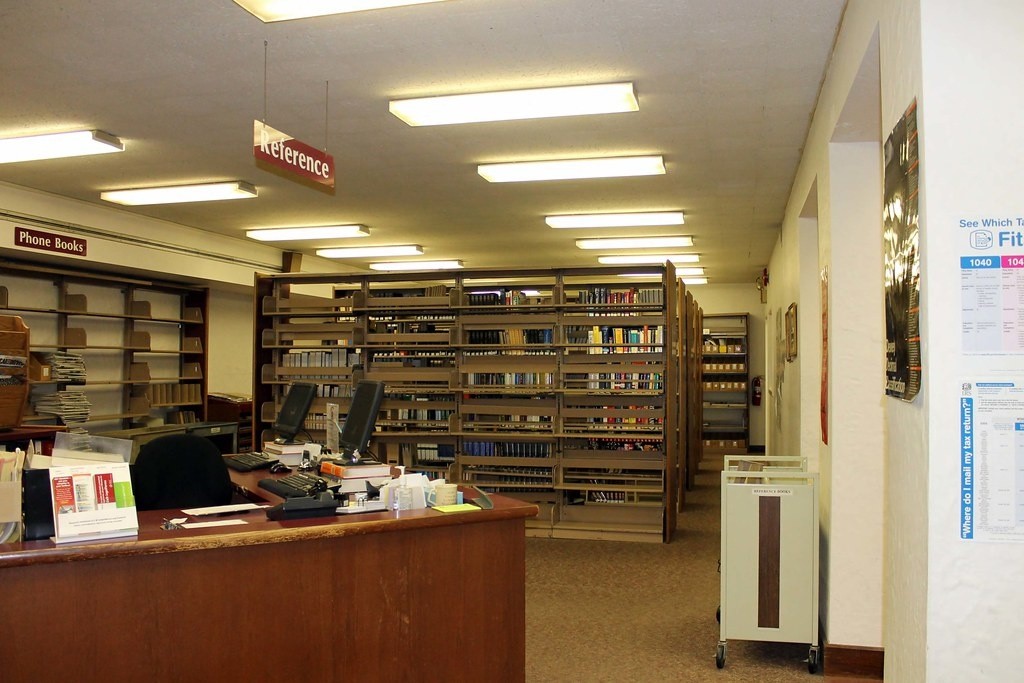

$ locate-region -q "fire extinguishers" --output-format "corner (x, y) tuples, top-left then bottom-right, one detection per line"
(752, 376), (762, 406)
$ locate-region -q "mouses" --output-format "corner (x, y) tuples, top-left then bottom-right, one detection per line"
(270, 462), (292, 473)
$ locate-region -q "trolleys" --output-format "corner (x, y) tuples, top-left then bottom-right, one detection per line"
(716, 454), (820, 675)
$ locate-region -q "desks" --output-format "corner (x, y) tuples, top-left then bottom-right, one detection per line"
(0, 455), (540, 683)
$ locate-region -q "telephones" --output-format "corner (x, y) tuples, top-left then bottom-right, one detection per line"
(266, 491), (339, 520)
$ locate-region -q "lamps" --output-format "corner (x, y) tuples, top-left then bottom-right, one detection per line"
(477, 155), (667, 184)
(545, 210), (686, 229)
(617, 266), (705, 278)
(0, 123), (127, 164)
(245, 224), (371, 242)
(369, 259), (464, 271)
(387, 81), (642, 130)
(575, 234), (694, 250)
(316, 244), (424, 257)
(597, 253), (702, 264)
(97, 180), (259, 207)
(681, 277), (710, 285)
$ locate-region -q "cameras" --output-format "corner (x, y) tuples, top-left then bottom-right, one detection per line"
(273, 381), (317, 445)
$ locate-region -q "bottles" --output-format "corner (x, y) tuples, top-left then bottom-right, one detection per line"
(392, 479), (413, 511)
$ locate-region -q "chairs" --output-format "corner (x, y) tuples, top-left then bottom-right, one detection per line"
(131, 434), (232, 512)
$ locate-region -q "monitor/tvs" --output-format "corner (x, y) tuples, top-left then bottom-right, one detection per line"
(332, 378), (385, 465)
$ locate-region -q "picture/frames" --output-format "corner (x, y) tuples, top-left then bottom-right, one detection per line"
(784, 301), (800, 362)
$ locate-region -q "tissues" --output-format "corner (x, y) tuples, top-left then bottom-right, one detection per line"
(422, 475), (463, 507)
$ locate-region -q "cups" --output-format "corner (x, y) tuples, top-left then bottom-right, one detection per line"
(427, 484), (458, 505)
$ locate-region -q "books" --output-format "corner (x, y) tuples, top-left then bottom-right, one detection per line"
(565, 286), (664, 503)
(417, 284), (456, 319)
(320, 462), (394, 492)
(376, 358), (455, 384)
(289, 318), (362, 346)
(313, 440), (326, 444)
(0, 355), (28, 387)
(43, 352), (86, 381)
(401, 444), (454, 467)
(463, 441), (553, 504)
(470, 291), (552, 344)
(0, 439), (139, 547)
(282, 349), (363, 380)
(339, 292), (393, 321)
(463, 350), (555, 428)
(283, 384), (356, 429)
(387, 393), (454, 432)
(264, 442), (305, 466)
(374, 324), (456, 356)
(24, 392), (92, 425)
(414, 470), (448, 489)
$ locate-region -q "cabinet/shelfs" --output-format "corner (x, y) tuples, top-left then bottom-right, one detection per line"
(0, 261), (210, 433)
(693, 299), (700, 476)
(331, 277), (686, 515)
(703, 310), (751, 453)
(208, 394), (252, 453)
(252, 258), (677, 544)
(686, 289), (695, 492)
(698, 307), (704, 463)
(717, 476), (805, 672)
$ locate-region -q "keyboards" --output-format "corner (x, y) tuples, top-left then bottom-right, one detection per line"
(228, 451), (280, 471)
(277, 472), (342, 495)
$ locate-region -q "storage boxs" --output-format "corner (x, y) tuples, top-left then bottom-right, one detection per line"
(701, 345), (742, 353)
(703, 440), (745, 448)
(703, 382), (746, 391)
(30, 352), (52, 381)
(702, 364), (745, 372)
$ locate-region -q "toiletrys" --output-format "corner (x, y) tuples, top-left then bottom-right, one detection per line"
(392, 465), (413, 510)
